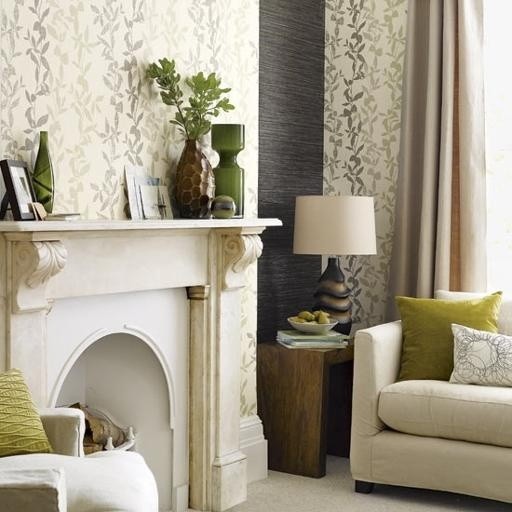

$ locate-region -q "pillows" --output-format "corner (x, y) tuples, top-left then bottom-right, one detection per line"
(393, 290), (504, 382)
(448, 323), (511, 387)
(0, 366), (53, 456)
(435, 291), (512, 336)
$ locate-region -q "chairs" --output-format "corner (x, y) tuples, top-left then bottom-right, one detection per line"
(0, 407), (159, 511)
(348, 318), (512, 504)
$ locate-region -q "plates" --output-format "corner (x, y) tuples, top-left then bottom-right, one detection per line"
(288, 316), (338, 333)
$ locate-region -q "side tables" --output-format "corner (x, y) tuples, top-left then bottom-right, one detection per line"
(256, 339), (354, 478)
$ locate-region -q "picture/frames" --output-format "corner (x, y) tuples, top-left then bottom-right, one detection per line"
(0, 159), (37, 221)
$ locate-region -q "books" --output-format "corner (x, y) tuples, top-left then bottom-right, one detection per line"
(276, 329), (348, 350)
(44, 212), (81, 221)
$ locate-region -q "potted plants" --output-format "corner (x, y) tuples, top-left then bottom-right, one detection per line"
(147, 57), (235, 219)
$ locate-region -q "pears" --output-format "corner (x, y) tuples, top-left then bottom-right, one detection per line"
(290, 310), (330, 325)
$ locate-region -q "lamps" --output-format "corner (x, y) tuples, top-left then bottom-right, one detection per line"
(292, 194), (376, 335)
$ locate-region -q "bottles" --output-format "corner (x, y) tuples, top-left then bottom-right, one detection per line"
(209, 123), (245, 218)
